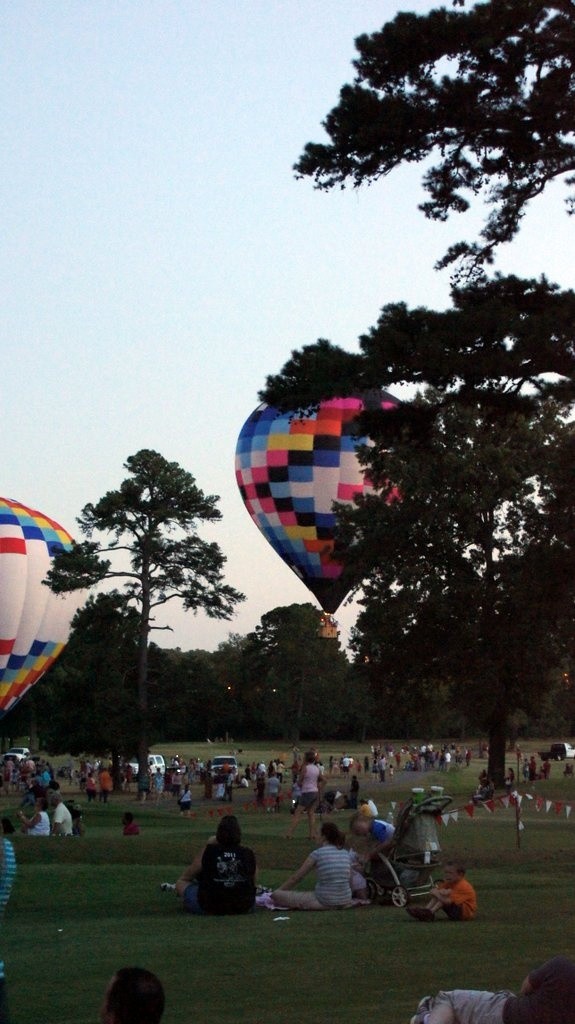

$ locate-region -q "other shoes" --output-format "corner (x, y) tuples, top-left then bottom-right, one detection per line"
(405, 906), (436, 921)
(409, 994), (433, 1024)
(160, 882), (176, 891)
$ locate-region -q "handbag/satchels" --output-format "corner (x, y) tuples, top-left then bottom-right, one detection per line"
(177, 799), (181, 805)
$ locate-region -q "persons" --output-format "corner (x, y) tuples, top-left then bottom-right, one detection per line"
(406, 860), (477, 921)
(100, 965), (166, 1024)
(271, 822), (352, 907)
(121, 812), (140, 835)
(0, 739), (575, 979)
(161, 815), (258, 916)
(410, 953), (575, 1024)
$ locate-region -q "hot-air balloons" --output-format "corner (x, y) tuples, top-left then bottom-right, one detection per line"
(0, 502), (88, 724)
(234, 385), (411, 639)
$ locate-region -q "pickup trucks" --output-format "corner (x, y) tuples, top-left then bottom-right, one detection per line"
(539, 741), (575, 762)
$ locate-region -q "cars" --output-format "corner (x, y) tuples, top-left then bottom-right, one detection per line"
(0, 747), (30, 761)
(127, 754), (165, 777)
(207, 755), (238, 781)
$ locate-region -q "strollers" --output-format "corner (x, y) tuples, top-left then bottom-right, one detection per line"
(356, 787), (454, 906)
